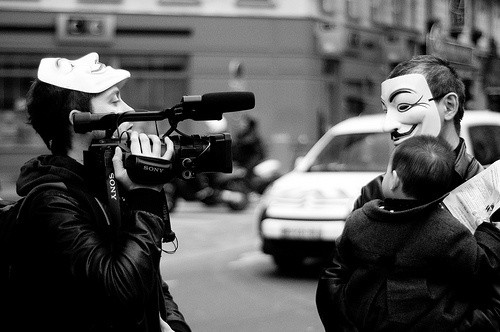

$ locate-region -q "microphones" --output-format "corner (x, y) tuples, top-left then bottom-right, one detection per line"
(181, 92), (255, 113)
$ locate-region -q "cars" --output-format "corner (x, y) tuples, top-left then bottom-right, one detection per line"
(254, 109), (500, 274)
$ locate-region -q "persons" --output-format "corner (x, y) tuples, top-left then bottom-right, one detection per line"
(0, 51), (193, 332)
(205, 116), (266, 166)
(338, 135), (500, 283)
(316, 54), (500, 332)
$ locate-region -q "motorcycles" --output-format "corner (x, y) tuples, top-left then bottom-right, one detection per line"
(165, 157), (248, 212)
(248, 159), (285, 196)
